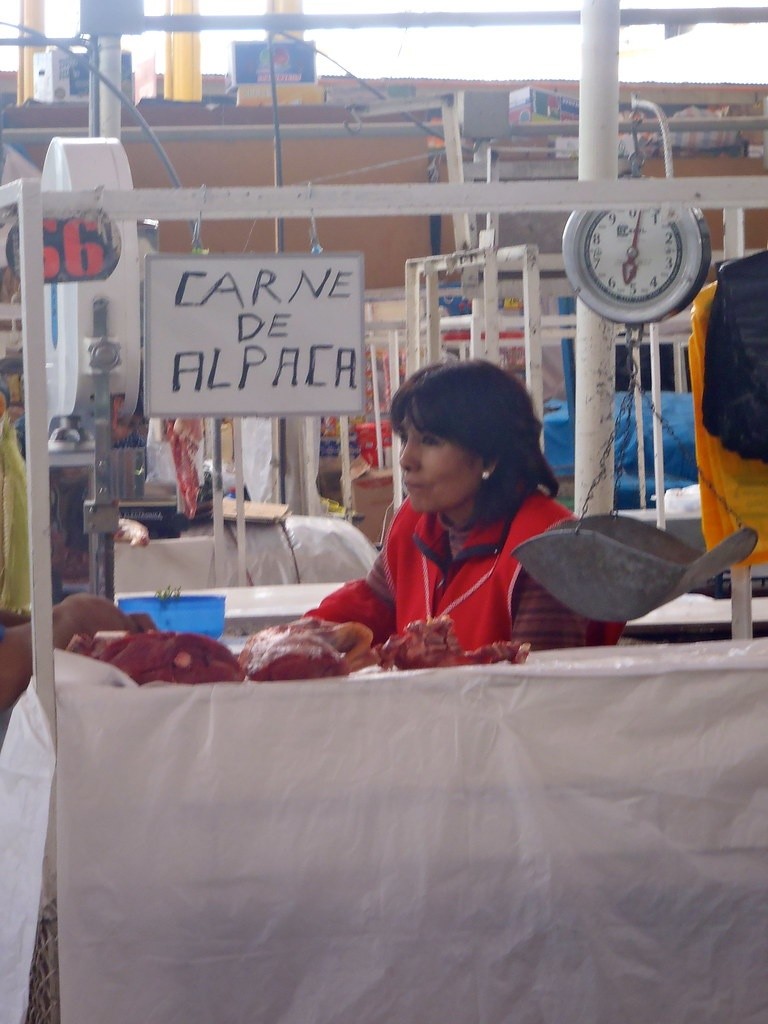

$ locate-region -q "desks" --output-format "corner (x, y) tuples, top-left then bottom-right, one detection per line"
(58, 507), (768, 1024)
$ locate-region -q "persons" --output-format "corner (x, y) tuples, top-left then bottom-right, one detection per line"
(0, 392), (181, 713)
(302, 360), (626, 653)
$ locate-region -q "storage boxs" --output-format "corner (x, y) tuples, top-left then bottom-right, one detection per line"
(337, 421), (419, 548)
(228, 39), (324, 106)
(508, 86), (579, 126)
